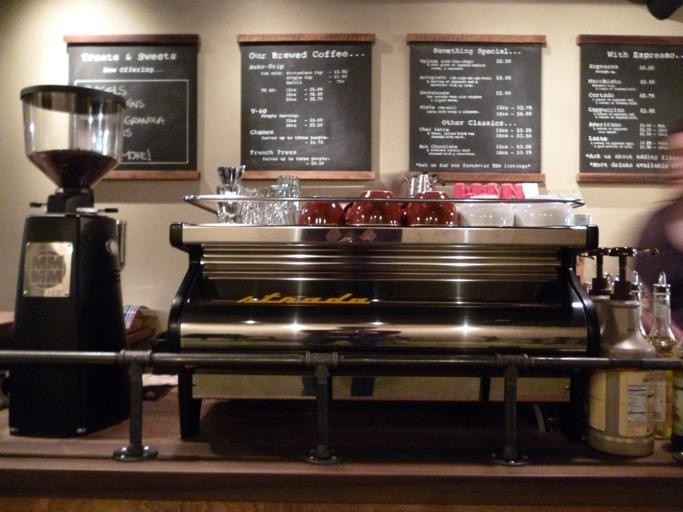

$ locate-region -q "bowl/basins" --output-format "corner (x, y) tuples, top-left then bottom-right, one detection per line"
(342, 190), (401, 226)
(514, 196), (573, 228)
(455, 195), (514, 228)
(401, 191), (459, 228)
(300, 196), (344, 226)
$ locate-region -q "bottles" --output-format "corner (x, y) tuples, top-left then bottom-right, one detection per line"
(585, 268), (683, 464)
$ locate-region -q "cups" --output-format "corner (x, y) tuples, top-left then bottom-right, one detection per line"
(451, 178), (539, 201)
(213, 161), (302, 228)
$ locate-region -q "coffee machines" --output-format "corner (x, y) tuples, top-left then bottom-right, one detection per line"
(9, 84), (130, 439)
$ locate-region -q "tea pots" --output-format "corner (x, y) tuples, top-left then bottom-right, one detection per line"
(400, 170), (444, 198)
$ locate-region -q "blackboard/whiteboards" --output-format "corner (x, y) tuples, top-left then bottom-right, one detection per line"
(405, 34), (547, 182)
(235, 33), (376, 181)
(577, 34), (683, 184)
(61, 34), (202, 180)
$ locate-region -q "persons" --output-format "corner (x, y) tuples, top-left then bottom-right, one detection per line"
(630, 130), (682, 338)
